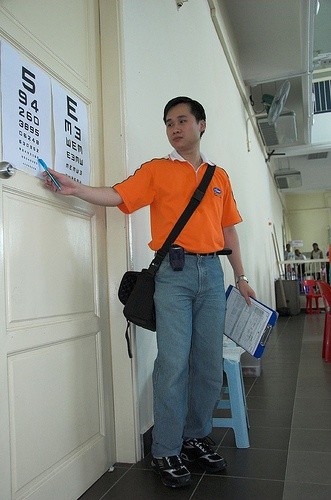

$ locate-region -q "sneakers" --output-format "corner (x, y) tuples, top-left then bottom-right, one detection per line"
(152, 454), (193, 488)
(180, 436), (226, 473)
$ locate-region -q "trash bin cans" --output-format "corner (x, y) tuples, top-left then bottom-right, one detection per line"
(276, 280), (301, 316)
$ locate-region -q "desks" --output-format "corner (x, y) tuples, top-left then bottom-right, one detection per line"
(283, 258), (329, 281)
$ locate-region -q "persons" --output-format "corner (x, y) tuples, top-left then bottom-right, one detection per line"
(310, 242), (330, 284)
(41, 94), (258, 490)
(284, 243), (294, 276)
(295, 250), (306, 277)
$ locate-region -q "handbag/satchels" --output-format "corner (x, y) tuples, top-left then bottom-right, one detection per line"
(118, 269), (156, 332)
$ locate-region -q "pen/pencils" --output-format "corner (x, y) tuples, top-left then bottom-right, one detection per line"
(38, 158), (62, 191)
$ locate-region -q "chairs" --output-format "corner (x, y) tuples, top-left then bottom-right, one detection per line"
(316, 280), (331, 362)
(304, 280), (322, 314)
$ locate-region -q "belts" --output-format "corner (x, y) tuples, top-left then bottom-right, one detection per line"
(157, 249), (232, 256)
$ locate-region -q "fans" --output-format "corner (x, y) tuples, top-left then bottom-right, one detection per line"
(262, 80), (290, 125)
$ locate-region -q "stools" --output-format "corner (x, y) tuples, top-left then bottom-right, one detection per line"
(212, 342), (250, 448)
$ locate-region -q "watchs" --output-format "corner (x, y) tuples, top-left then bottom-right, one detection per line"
(234, 274), (250, 284)
